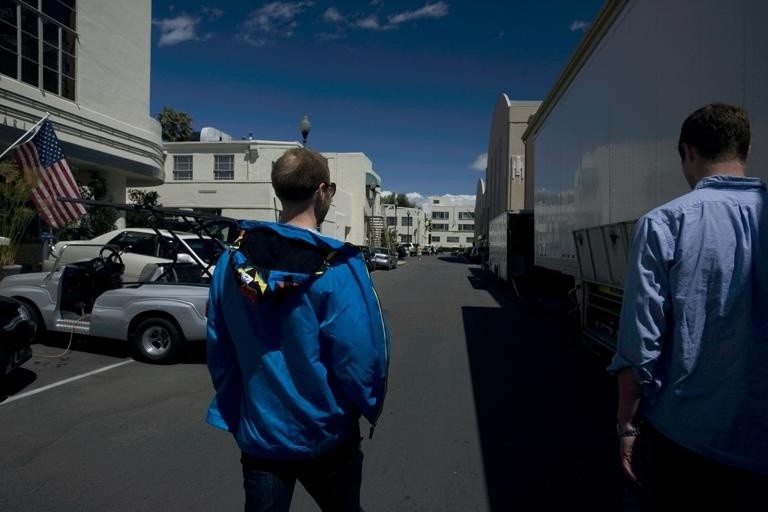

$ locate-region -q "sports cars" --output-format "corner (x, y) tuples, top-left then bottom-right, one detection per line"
(1, 196), (238, 362)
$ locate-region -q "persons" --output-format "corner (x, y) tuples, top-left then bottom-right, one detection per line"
(607, 101), (768, 511)
(202, 146), (393, 512)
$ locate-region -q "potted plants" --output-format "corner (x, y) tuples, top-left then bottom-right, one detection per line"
(0, 159), (50, 281)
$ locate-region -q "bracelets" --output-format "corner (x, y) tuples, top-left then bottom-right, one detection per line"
(614, 422), (643, 437)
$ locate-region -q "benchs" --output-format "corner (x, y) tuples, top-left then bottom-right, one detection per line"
(127, 262), (192, 288)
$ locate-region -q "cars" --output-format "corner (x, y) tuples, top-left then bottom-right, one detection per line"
(422, 245), (437, 256)
(43, 225), (227, 284)
(1, 295), (37, 377)
(371, 247), (398, 269)
(401, 243), (417, 256)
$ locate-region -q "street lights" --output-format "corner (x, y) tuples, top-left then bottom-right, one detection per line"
(394, 201), (398, 230)
(407, 211), (411, 242)
(301, 116), (310, 148)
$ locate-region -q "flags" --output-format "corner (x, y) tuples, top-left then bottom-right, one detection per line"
(10, 117), (86, 230)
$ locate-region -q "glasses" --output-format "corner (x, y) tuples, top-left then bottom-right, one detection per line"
(320, 182), (336, 197)
